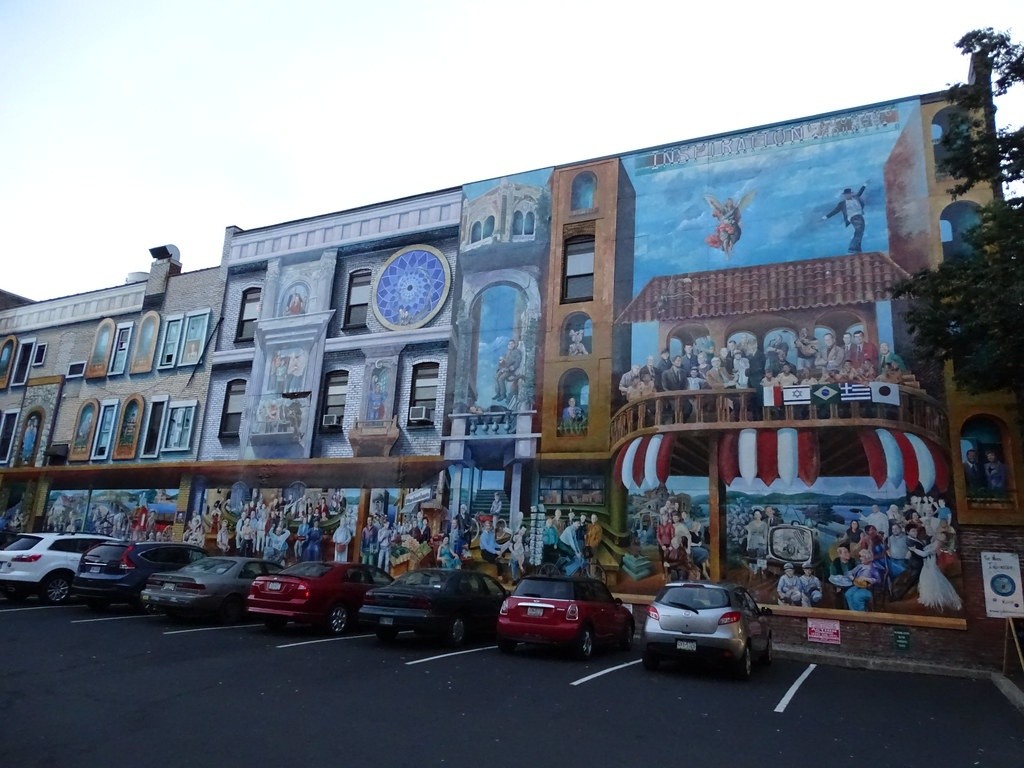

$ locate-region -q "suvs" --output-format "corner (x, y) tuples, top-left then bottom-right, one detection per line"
(70, 541), (210, 610)
(0, 531), (122, 606)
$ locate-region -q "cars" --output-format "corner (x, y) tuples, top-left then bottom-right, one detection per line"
(140, 556), (288, 626)
(640, 580), (774, 684)
(496, 572), (636, 661)
(358, 567), (510, 648)
(245, 560), (394, 637)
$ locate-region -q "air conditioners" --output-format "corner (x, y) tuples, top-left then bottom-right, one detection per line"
(322, 414), (337, 426)
(409, 406), (431, 422)
